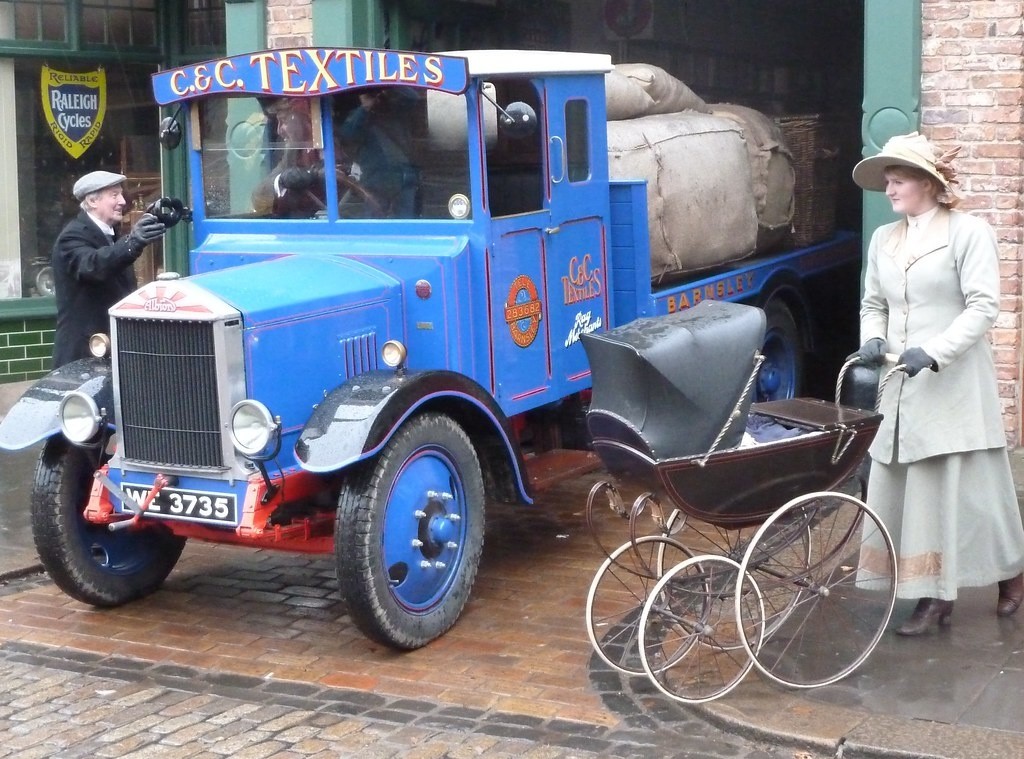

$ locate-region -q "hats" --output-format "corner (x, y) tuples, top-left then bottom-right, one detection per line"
(72, 171), (127, 201)
(853, 132), (964, 209)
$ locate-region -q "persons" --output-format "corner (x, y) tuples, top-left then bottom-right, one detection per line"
(245, 92), (365, 219)
(850, 131), (1024, 635)
(50, 172), (166, 373)
(338, 87), (423, 220)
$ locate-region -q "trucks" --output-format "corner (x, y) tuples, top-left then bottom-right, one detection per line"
(0, 49), (862, 650)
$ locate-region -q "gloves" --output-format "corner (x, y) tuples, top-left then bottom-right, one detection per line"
(845, 338), (886, 370)
(311, 160), (351, 183)
(896, 347), (935, 377)
(127, 213), (166, 257)
(279, 166), (311, 191)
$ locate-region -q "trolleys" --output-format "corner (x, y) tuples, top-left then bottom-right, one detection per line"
(579, 300), (906, 704)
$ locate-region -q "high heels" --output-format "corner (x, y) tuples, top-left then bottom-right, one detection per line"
(997, 573), (1024, 616)
(896, 594), (954, 636)
